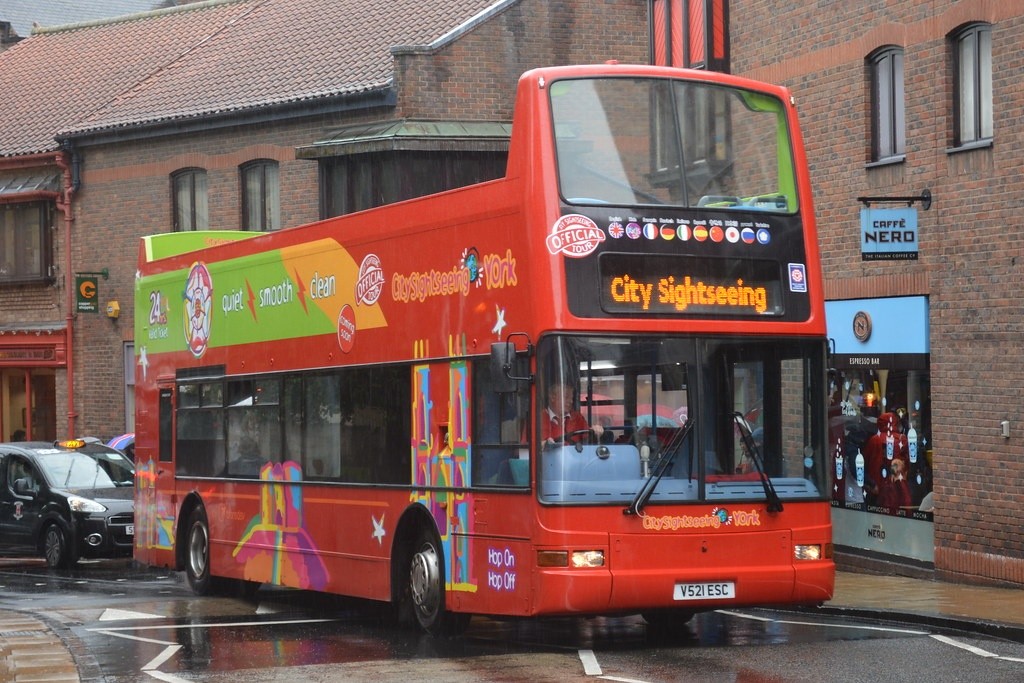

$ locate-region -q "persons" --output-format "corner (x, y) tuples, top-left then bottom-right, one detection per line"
(843, 413), (912, 516)
(228, 435), (265, 476)
(13, 429), (27, 442)
(125, 443), (135, 465)
(736, 426), (789, 479)
(21, 460), (40, 490)
(520, 368), (604, 447)
(596, 417), (614, 444)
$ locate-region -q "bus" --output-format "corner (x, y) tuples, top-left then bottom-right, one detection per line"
(132, 59), (839, 637)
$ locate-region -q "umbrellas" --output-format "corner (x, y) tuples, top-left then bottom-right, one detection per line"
(828, 414), (880, 433)
(106, 433), (134, 450)
(579, 392), (752, 436)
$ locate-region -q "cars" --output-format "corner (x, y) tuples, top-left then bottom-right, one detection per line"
(0, 440), (140, 570)
